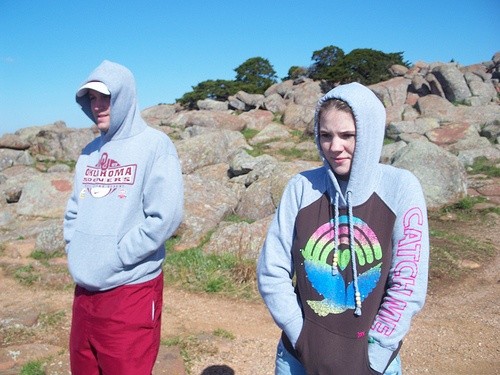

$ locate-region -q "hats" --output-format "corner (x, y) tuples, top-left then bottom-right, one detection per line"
(76, 81), (110, 97)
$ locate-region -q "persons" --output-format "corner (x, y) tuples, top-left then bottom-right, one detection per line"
(257, 82), (429, 375)
(63, 61), (182, 375)
